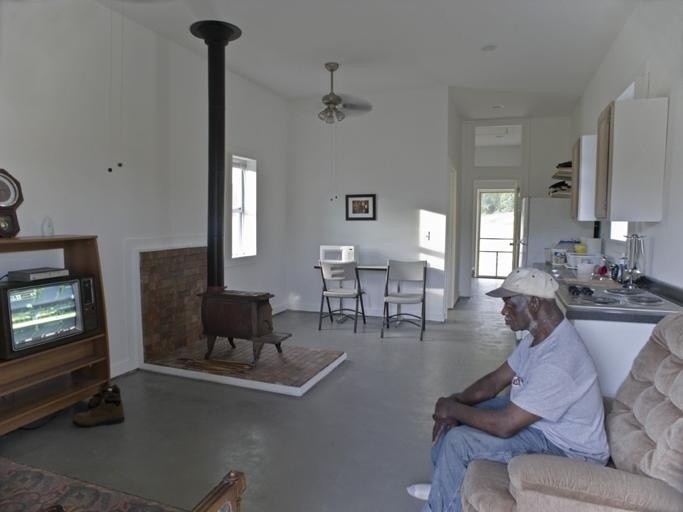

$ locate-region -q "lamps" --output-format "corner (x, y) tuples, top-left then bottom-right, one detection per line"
(317, 107), (344, 124)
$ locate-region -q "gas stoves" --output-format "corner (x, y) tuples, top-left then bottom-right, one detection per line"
(558, 279), (683, 320)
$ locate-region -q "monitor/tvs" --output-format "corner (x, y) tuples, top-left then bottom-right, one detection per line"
(0, 272), (101, 361)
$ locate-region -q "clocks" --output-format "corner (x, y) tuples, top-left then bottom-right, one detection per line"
(1, 169), (24, 239)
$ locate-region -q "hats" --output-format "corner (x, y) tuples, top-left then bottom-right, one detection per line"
(485, 267), (560, 299)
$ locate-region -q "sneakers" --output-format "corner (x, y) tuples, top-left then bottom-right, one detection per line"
(72, 392), (125, 428)
(88, 384), (120, 408)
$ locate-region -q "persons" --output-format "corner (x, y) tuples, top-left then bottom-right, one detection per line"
(406, 266), (610, 512)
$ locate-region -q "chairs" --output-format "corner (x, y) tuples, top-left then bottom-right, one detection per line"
(318, 259), (366, 333)
(380, 259), (427, 341)
(459, 310), (683, 512)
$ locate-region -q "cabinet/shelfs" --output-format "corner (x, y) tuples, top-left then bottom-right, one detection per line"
(570, 137), (596, 221)
(0, 234), (110, 437)
(548, 167), (572, 198)
(594, 97), (671, 221)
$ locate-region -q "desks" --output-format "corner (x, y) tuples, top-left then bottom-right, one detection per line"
(314, 264), (387, 323)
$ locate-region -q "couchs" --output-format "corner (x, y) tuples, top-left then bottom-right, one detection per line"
(1, 455), (246, 512)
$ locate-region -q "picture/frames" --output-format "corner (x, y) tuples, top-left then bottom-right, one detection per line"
(345, 195), (376, 221)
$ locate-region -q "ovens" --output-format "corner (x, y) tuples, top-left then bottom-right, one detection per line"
(320, 245), (360, 265)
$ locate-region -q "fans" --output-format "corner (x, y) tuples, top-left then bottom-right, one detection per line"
(288, 89), (372, 119)
